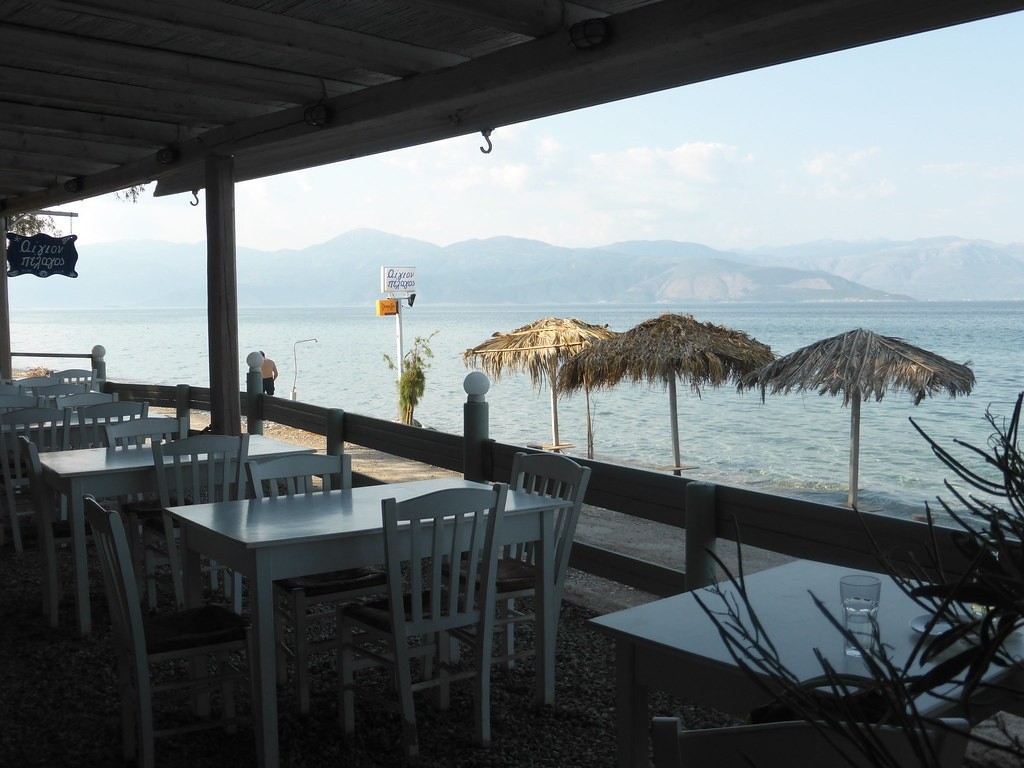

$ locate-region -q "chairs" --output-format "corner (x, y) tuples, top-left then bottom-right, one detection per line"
(244, 453), (389, 718)
(0, 406), (72, 560)
(651, 716), (971, 768)
(443, 451), (592, 666)
(19, 435), (126, 630)
(76, 401), (149, 449)
(105, 416), (194, 577)
(82, 492), (253, 768)
(0, 369), (119, 414)
(336, 482), (510, 761)
(141, 431), (250, 609)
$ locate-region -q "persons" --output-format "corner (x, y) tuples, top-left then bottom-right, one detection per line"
(258, 351), (279, 397)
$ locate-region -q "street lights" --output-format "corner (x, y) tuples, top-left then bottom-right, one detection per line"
(387, 293), (417, 421)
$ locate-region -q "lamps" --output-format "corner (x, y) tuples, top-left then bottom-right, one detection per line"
(157, 148), (180, 165)
(63, 178), (85, 193)
(568, 18), (614, 51)
(304, 104), (335, 127)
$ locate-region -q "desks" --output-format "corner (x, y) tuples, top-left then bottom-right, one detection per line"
(37, 434), (317, 635)
(0, 416), (164, 547)
(163, 475), (574, 768)
(585, 557), (1024, 768)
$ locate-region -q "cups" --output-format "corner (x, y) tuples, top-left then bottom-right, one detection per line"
(839, 575), (881, 614)
(844, 615), (877, 657)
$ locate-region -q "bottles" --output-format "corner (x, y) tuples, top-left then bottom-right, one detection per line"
(972, 533), (998, 614)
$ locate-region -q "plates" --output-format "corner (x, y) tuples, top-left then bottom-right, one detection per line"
(909, 613), (979, 639)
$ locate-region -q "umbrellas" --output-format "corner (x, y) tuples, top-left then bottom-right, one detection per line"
(465, 315), (627, 453)
(736, 326), (976, 509)
(558, 311), (774, 477)
